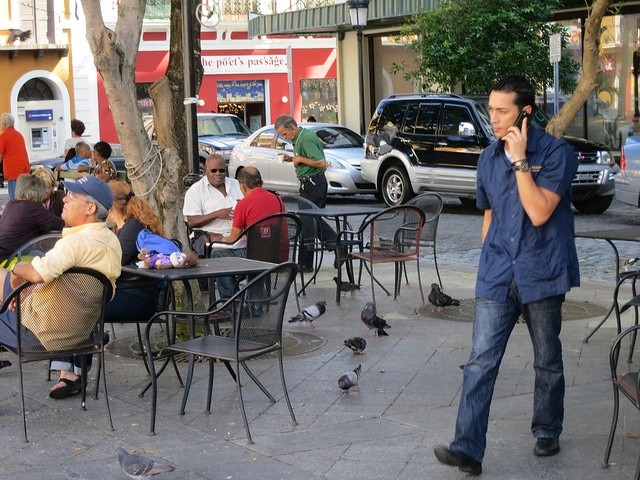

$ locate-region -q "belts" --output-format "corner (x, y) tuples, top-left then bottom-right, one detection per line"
(300, 172), (325, 182)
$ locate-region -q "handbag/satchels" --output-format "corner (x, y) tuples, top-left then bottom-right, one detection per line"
(136, 229), (181, 255)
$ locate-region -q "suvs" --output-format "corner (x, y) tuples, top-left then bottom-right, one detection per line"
(360, 93), (618, 212)
(197, 113), (252, 176)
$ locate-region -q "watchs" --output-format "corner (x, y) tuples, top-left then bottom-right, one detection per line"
(512, 161), (531, 172)
(49, 178), (170, 400)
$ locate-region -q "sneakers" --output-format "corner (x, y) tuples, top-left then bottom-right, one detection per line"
(262, 289), (278, 304)
(236, 300), (263, 318)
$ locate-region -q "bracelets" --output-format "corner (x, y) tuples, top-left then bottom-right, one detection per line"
(11, 262), (17, 276)
(511, 159), (529, 166)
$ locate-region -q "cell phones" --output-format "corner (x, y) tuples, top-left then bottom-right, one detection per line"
(513, 110), (533, 132)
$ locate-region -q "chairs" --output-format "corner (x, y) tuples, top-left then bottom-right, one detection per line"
(603, 325), (638, 480)
(272, 196), (354, 293)
(336, 207), (427, 322)
(1, 235), (60, 380)
(357, 193), (444, 296)
(612, 275), (640, 367)
(184, 221), (213, 313)
(0, 267), (116, 436)
(53, 166), (127, 191)
(204, 213), (303, 319)
(144, 263), (301, 437)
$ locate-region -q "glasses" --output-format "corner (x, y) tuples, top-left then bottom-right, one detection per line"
(211, 169), (225, 172)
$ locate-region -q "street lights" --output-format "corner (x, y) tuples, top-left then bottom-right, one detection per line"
(347, 0), (368, 135)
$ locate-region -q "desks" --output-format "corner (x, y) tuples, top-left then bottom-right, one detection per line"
(574, 228), (640, 342)
(288, 209), (394, 296)
(123, 256), (283, 413)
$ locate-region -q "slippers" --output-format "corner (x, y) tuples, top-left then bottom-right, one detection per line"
(50, 377), (81, 399)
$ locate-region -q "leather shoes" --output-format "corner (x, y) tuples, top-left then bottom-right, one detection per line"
(334, 245), (348, 269)
(296, 263), (313, 272)
(534, 438), (560, 457)
(434, 446), (482, 475)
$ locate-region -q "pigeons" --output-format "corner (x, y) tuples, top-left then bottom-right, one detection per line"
(344, 337), (366, 354)
(332, 277), (360, 297)
(288, 300), (327, 328)
(338, 364), (362, 392)
(428, 283), (460, 313)
(361, 302), (391, 338)
(113, 446), (176, 480)
(615, 258), (640, 272)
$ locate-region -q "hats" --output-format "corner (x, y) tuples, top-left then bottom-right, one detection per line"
(61, 176), (114, 210)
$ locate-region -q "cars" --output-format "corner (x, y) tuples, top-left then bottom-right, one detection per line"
(614, 136), (640, 207)
(227, 124), (383, 203)
(29, 144), (124, 172)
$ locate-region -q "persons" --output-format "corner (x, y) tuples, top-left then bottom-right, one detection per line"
(434, 76), (580, 475)
(211, 168), (289, 315)
(306, 116), (318, 123)
(54, 142), (97, 174)
(183, 154), (280, 316)
(75, 141), (118, 181)
(34, 167), (64, 218)
(0, 114), (31, 201)
(0, 174), (65, 264)
(273, 115), (347, 273)
(62, 118), (92, 155)
(0, 174), (123, 355)
(138, 247), (199, 268)
